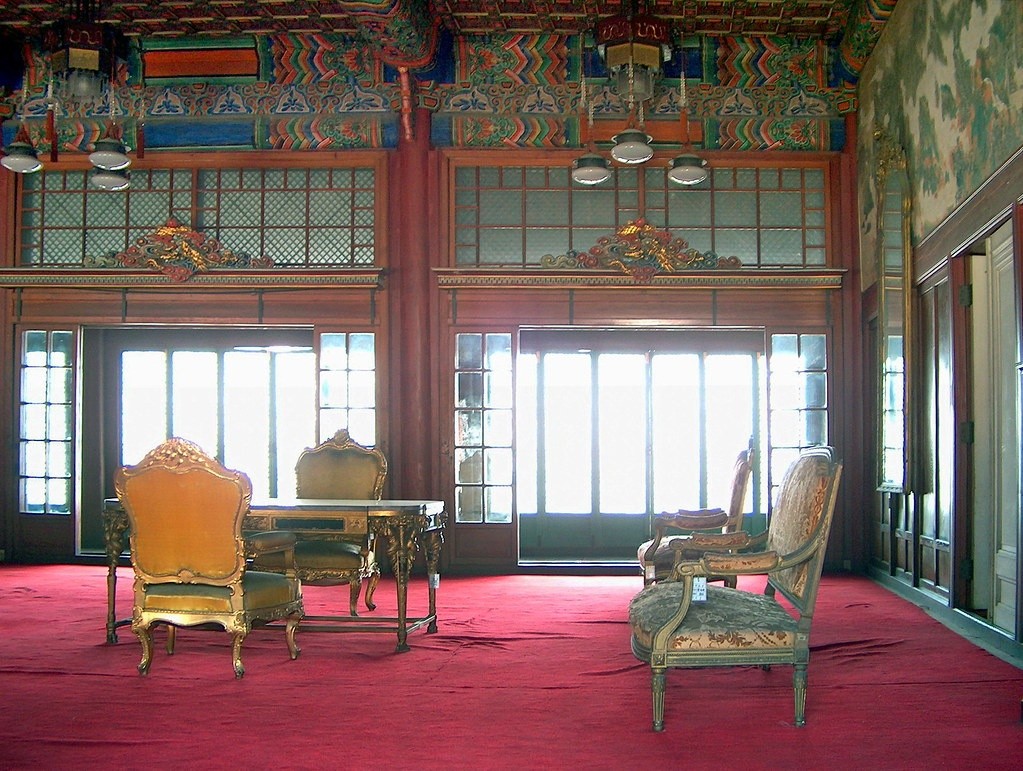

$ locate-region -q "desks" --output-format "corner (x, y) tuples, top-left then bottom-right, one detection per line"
(101, 496), (447, 653)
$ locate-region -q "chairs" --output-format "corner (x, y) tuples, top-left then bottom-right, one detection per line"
(626, 446), (846, 734)
(253, 428), (386, 619)
(113, 435), (303, 683)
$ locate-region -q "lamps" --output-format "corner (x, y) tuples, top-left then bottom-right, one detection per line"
(570, 0), (709, 186)
(0, 0), (133, 192)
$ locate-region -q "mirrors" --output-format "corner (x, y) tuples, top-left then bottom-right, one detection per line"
(873, 121), (914, 495)
